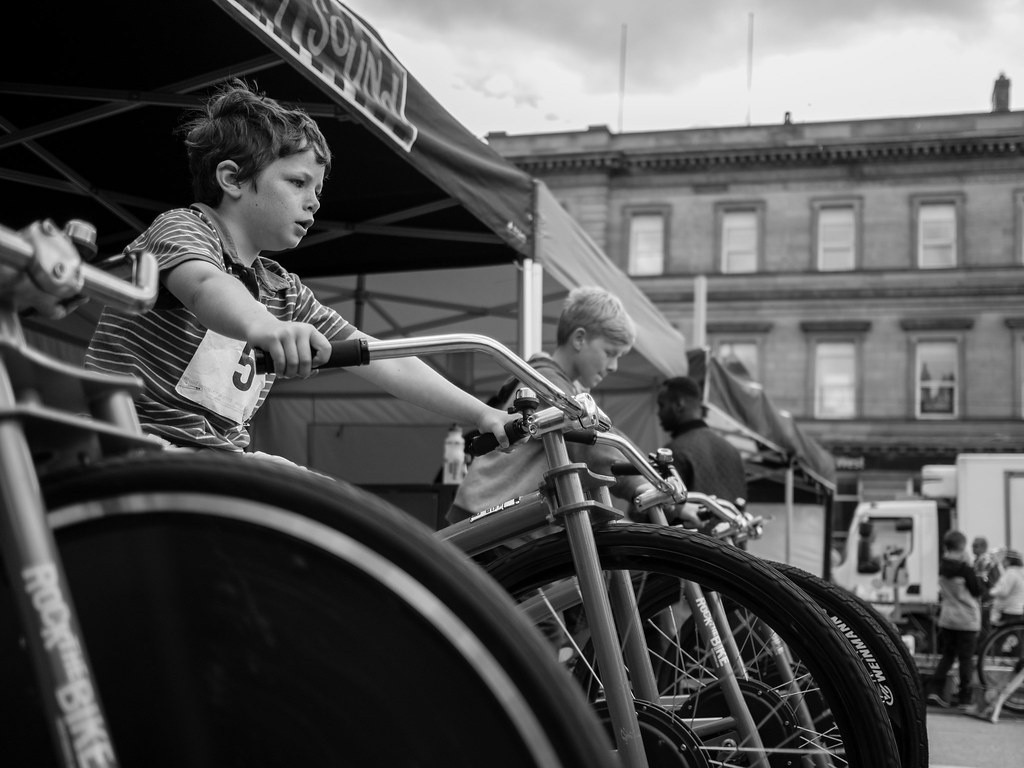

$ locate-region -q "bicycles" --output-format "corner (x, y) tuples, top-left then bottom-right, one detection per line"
(978, 614), (1024, 717)
(0, 213), (929, 768)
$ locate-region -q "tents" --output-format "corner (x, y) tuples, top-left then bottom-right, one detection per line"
(0, 0), (839, 582)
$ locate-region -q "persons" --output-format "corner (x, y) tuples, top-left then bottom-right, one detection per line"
(928, 530), (987, 709)
(988, 549), (1024, 656)
(79, 76), (523, 458)
(448, 286), (686, 697)
(973, 537), (995, 604)
(646, 376), (748, 695)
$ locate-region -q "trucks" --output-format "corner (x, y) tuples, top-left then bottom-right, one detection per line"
(830, 452), (1023, 663)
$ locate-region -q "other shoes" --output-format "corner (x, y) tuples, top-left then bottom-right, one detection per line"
(958, 699), (978, 712)
(927, 691), (950, 708)
